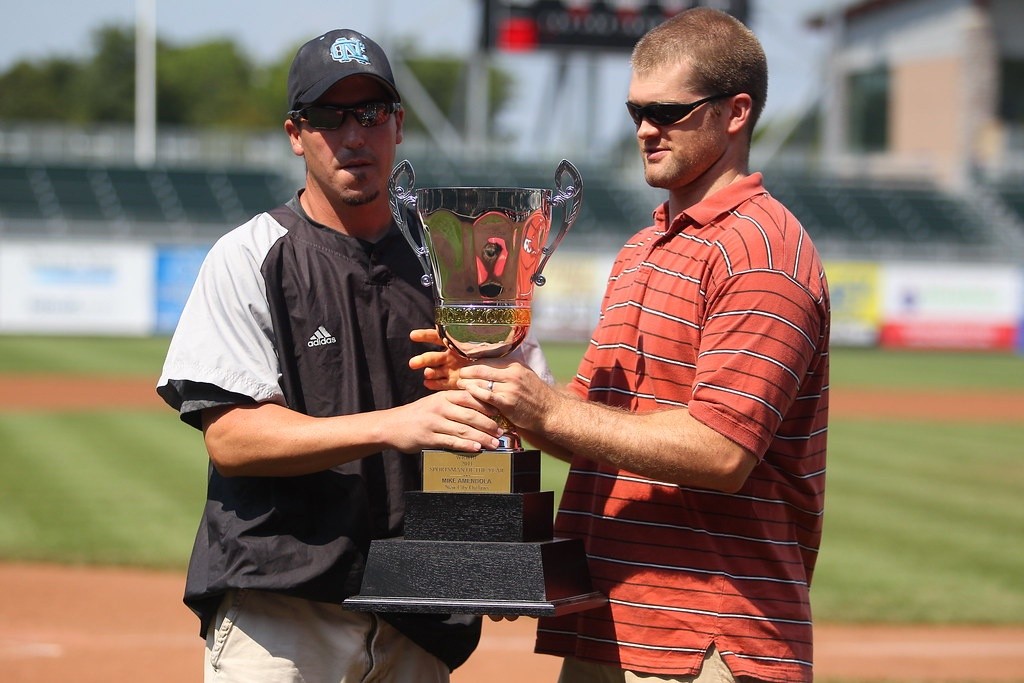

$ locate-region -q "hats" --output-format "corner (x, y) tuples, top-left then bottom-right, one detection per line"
(286, 29), (401, 118)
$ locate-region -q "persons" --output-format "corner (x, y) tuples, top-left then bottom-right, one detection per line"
(155, 29), (557, 683)
(407, 8), (831, 683)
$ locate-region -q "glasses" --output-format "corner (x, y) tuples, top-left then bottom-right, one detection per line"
(290, 100), (398, 129)
(625, 91), (737, 126)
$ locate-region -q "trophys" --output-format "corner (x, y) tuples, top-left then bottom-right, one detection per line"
(343, 156), (608, 615)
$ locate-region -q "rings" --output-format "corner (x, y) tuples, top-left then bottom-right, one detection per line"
(489, 381), (493, 392)
